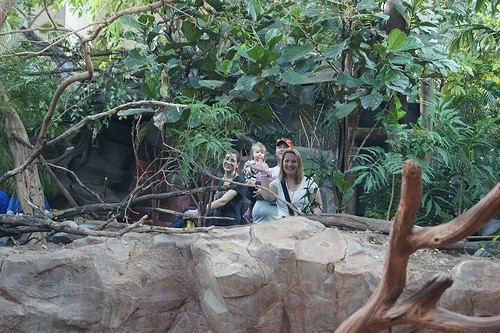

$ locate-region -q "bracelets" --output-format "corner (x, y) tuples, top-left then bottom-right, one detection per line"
(260, 172), (270, 178)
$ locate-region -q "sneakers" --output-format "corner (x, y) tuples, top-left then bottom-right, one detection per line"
(243, 213), (253, 223)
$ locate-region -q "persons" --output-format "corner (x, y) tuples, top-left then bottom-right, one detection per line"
(250, 148), (324, 219)
(183, 149), (246, 226)
(252, 138), (293, 224)
(0, 189), (50, 216)
(243, 141), (273, 223)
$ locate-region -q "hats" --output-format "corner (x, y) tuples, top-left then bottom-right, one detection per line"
(276, 137), (292, 147)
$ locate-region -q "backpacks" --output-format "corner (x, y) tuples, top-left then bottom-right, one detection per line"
(170, 207), (197, 228)
